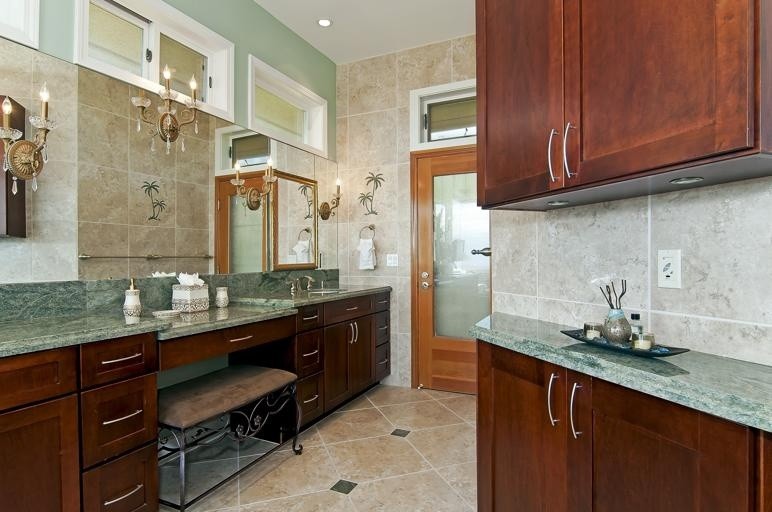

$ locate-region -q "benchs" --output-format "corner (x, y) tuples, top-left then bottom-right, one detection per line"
(156, 361), (306, 510)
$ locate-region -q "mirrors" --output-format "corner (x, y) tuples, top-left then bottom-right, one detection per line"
(273, 168), (320, 271)
(74, 0), (156, 85)
(247, 53), (329, 159)
(0, 36), (337, 285)
(157, 0), (236, 124)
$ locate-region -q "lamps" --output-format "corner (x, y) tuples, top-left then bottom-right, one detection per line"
(229, 156), (253, 212)
(0, 78), (59, 196)
(0, 95), (23, 160)
(129, 83), (157, 157)
(157, 65), (203, 157)
(319, 176), (343, 220)
(247, 156), (276, 211)
(131, 58), (210, 158)
(7, 80), (53, 179)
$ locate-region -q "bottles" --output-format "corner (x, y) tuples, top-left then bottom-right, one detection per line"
(123, 288), (143, 325)
(630, 314), (642, 335)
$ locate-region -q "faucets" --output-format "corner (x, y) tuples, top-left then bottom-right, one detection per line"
(297, 275), (316, 292)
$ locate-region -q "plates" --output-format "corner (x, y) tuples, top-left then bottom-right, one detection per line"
(153, 310), (181, 316)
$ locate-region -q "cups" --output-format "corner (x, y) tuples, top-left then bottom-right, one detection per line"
(633, 333), (657, 352)
(215, 286), (229, 306)
(584, 323), (602, 339)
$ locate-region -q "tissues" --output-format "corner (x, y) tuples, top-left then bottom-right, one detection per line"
(171, 271), (210, 314)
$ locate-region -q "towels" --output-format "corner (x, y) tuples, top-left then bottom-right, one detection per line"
(289, 238), (315, 266)
(354, 235), (377, 270)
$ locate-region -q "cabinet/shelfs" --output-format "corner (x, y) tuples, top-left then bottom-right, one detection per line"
(324, 292), (372, 414)
(230, 305), (324, 432)
(755, 429), (772, 511)
(156, 309), (299, 372)
(475, 0), (772, 216)
(372, 286), (393, 386)
(475, 337), (753, 511)
(80, 330), (159, 512)
(0, 347), (81, 511)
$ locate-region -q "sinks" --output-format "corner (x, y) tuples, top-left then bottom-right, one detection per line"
(296, 288), (341, 298)
